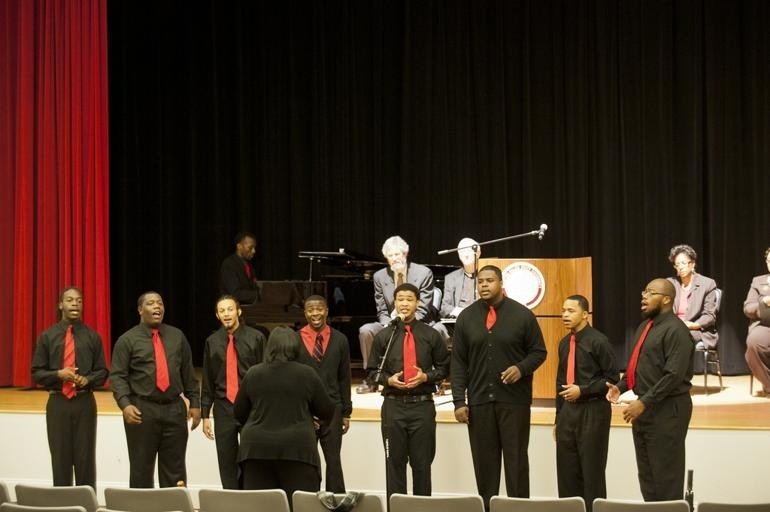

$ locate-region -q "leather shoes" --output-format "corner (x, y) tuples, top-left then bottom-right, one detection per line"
(356, 383), (373, 394)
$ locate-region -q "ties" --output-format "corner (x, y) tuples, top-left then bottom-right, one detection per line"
(397, 273), (403, 286)
(62, 325), (78, 400)
(313, 335), (325, 363)
(403, 326), (418, 384)
(245, 265), (251, 279)
(566, 335), (576, 386)
(226, 333), (240, 404)
(152, 329), (171, 393)
(627, 320), (654, 390)
(487, 304), (496, 330)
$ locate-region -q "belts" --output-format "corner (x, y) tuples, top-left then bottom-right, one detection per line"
(383, 394), (433, 403)
(49, 388), (87, 396)
(134, 392), (172, 405)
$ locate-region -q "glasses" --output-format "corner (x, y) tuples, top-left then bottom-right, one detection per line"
(641, 291), (666, 297)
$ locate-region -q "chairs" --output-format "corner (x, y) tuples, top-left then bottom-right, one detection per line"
(591, 497), (690, 511)
(694, 288), (723, 395)
(389, 493), (485, 512)
(697, 502), (770, 512)
(490, 496), (586, 512)
(748, 319), (762, 395)
(0, 483), (196, 512)
(433, 286), (442, 311)
(198, 488), (291, 512)
(292, 490), (385, 512)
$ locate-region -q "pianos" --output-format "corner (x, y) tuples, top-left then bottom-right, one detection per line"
(239, 251), (462, 361)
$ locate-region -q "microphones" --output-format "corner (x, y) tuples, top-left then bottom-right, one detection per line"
(388, 313), (405, 326)
(538, 223), (548, 241)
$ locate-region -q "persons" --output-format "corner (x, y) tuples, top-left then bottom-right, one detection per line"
(365, 283), (449, 512)
(665, 244), (722, 372)
(31, 288), (109, 495)
(201, 292), (353, 512)
(605, 278), (695, 501)
(743, 248), (770, 397)
(431, 238), (482, 395)
(449, 266), (547, 512)
(221, 233), (258, 304)
(355, 236), (435, 395)
(555, 295), (620, 512)
(109, 290), (202, 488)
